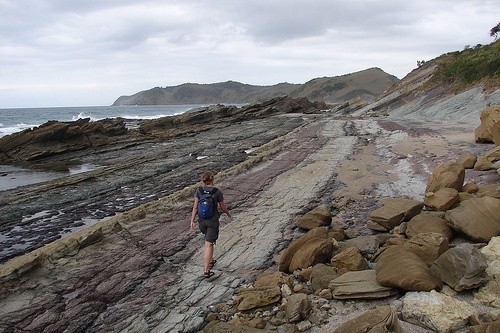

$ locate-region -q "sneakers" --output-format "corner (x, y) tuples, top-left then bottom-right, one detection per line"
(204, 270), (214, 278)
(209, 260), (217, 268)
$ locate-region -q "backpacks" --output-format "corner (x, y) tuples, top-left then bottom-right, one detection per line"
(198, 186), (219, 219)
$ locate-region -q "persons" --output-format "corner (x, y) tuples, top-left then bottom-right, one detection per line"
(190, 171), (231, 276)
